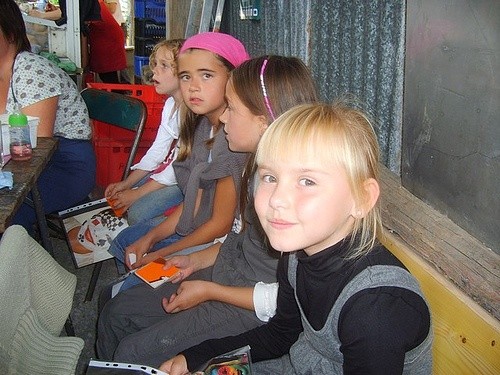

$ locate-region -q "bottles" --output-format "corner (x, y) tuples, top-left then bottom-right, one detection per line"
(8, 102), (34, 162)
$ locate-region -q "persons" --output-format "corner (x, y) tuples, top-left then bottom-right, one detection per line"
(93, 55), (320, 374)
(108, 32), (249, 272)
(153, 102), (435, 375)
(26, 0), (129, 84)
(104, 38), (190, 220)
(0, 0), (97, 233)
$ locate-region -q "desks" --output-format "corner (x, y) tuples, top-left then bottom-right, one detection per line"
(1, 135), (77, 344)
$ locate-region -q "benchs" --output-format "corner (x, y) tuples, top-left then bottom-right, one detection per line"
(368, 160), (500, 375)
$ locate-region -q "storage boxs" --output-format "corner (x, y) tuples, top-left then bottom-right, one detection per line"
(85, 82), (169, 142)
(132, 56), (149, 76)
(134, 36), (163, 56)
(134, 18), (166, 40)
(92, 141), (156, 190)
(133, 0), (167, 23)
(134, 76), (142, 84)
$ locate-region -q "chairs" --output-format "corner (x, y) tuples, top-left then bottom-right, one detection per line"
(70, 89), (147, 302)
